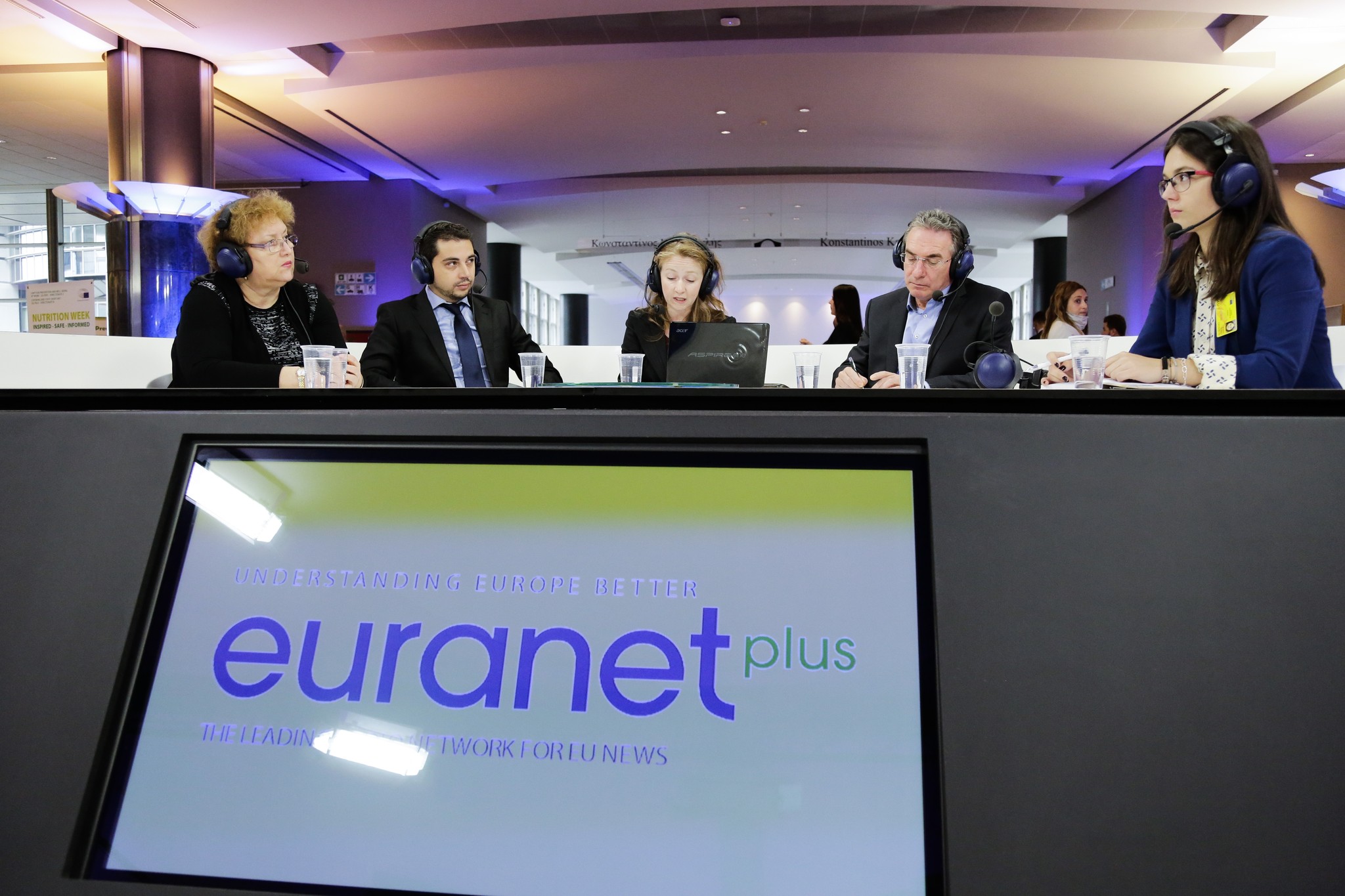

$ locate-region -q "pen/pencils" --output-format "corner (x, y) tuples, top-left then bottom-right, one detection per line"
(1026, 349), (1088, 373)
(848, 356), (865, 388)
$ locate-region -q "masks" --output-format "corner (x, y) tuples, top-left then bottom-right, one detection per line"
(1064, 312), (1089, 330)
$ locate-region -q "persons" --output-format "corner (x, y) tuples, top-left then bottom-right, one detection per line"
(1040, 115), (1343, 388)
(359, 220), (563, 387)
(1040, 280), (1089, 339)
(616, 231), (738, 383)
(799, 284), (863, 344)
(830, 208), (1013, 389)
(1029, 311), (1046, 339)
(168, 190), (364, 389)
(1101, 314), (1127, 337)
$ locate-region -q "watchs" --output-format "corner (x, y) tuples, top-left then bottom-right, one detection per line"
(295, 366), (306, 389)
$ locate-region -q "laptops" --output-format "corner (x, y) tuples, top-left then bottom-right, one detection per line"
(669, 321), (771, 388)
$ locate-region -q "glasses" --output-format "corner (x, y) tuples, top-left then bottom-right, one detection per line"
(900, 252), (952, 270)
(243, 235), (298, 254)
(1158, 171), (1214, 198)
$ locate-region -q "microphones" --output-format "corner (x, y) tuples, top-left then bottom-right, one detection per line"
(1163, 179), (1253, 239)
(473, 269), (488, 294)
(644, 281), (665, 314)
(295, 257), (308, 274)
(932, 265), (975, 301)
(989, 302), (1005, 352)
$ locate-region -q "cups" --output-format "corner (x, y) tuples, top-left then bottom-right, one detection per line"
(518, 353), (547, 388)
(895, 344), (931, 389)
(1069, 334), (1111, 390)
(618, 354), (645, 382)
(329, 348), (349, 388)
(792, 351), (822, 389)
(301, 345), (335, 388)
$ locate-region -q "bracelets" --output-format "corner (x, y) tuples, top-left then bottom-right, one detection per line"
(1170, 356), (1178, 385)
(1168, 357), (1173, 384)
(321, 374), (324, 388)
(1161, 356), (1170, 384)
(1177, 357), (1188, 386)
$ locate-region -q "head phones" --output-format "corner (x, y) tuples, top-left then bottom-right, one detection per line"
(963, 342), (1050, 389)
(892, 212), (974, 284)
(215, 196), (254, 279)
(1176, 121), (1263, 207)
(409, 219), (480, 285)
(647, 235), (718, 300)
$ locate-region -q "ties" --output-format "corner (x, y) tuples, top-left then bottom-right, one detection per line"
(439, 301), (487, 387)
